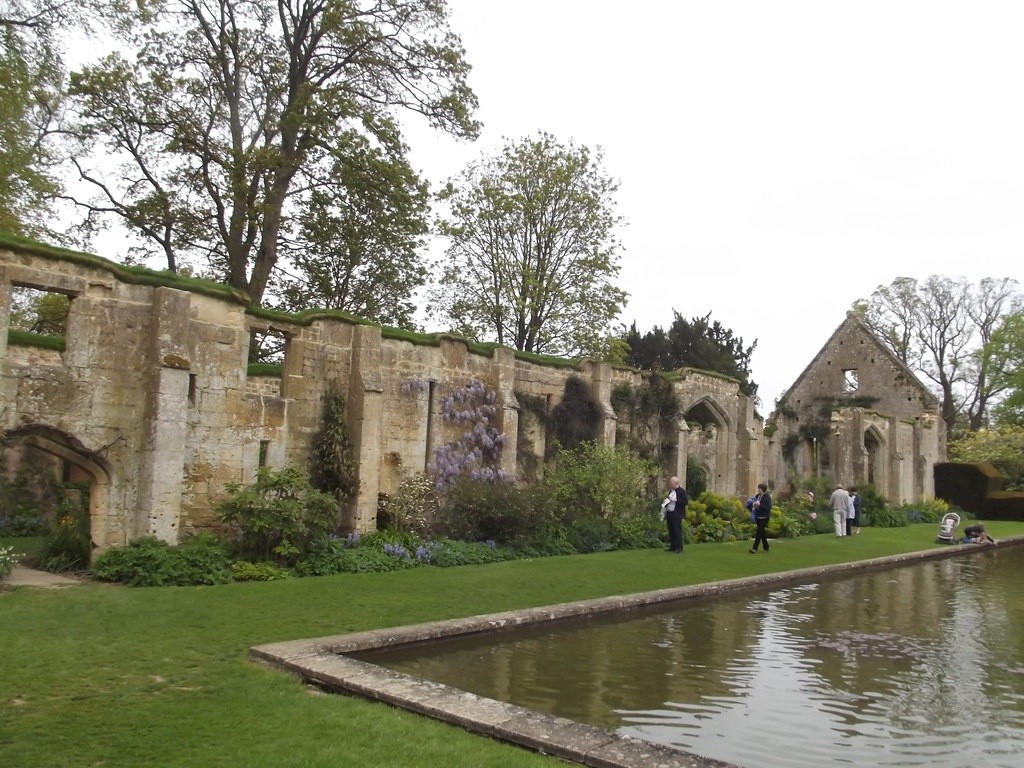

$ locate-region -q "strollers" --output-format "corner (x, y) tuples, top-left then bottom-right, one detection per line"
(935, 513), (961, 545)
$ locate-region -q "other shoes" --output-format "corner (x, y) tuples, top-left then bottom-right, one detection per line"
(749, 549), (757, 553)
(664, 549), (676, 552)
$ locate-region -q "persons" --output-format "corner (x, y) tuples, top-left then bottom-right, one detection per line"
(963, 522), (998, 550)
(828, 483), (849, 538)
(843, 490), (856, 537)
(850, 486), (862, 535)
(663, 476), (688, 554)
(748, 483), (772, 554)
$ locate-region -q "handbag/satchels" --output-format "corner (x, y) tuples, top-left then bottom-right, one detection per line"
(661, 506), (666, 521)
